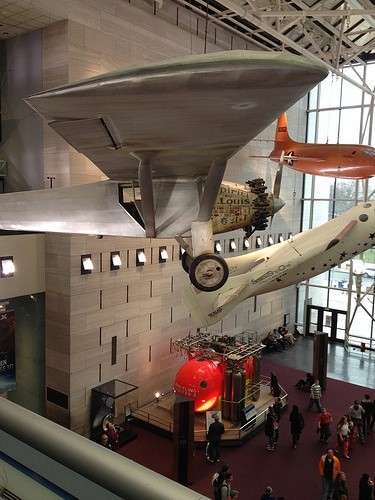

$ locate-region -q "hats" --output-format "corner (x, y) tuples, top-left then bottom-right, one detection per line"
(354, 400), (359, 405)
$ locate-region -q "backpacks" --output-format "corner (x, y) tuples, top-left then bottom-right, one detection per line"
(211, 472), (220, 487)
(214, 483), (228, 500)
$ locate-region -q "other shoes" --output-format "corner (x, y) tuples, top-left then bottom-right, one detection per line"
(294, 339), (298, 341)
(206, 456), (214, 462)
(215, 459), (220, 462)
(344, 455), (350, 459)
(334, 449), (339, 453)
(265, 444), (272, 446)
(267, 447), (274, 451)
(294, 445), (296, 448)
(273, 440), (279, 445)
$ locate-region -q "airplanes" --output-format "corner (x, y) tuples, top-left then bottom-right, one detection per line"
(248, 114), (373, 181)
(0, 48), (286, 293)
(179, 201), (375, 330)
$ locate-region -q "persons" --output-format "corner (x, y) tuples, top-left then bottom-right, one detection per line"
(267, 325), (299, 352)
(204, 372), (375, 500)
(99, 423), (119, 451)
